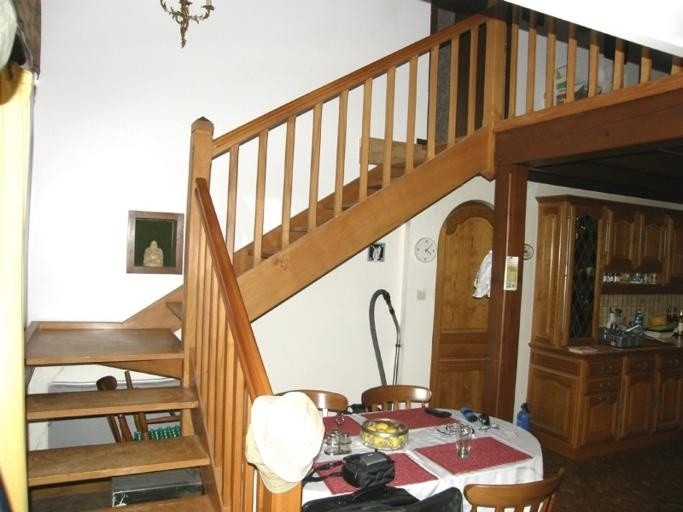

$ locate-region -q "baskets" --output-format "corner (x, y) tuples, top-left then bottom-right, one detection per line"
(598, 326), (644, 348)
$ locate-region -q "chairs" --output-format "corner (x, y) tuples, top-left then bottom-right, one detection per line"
(363, 386), (433, 412)
(464, 467), (566, 512)
(97, 376), (182, 443)
(274, 390), (348, 416)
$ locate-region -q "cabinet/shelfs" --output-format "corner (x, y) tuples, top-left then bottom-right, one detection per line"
(527, 194), (683, 461)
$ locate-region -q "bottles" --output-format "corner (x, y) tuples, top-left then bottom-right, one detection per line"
(326, 428), (351, 455)
(666, 304), (683, 336)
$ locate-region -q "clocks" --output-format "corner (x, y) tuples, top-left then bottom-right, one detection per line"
(415, 237), (437, 264)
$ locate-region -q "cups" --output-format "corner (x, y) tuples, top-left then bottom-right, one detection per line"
(453, 428), (473, 459)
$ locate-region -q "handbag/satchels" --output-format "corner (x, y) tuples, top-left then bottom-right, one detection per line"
(341, 449), (395, 489)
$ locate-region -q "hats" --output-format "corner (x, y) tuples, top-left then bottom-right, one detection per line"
(243, 391), (326, 494)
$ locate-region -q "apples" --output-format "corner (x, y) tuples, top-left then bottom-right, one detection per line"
(368, 422), (402, 448)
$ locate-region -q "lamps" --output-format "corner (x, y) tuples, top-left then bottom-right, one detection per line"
(160, 0), (214, 47)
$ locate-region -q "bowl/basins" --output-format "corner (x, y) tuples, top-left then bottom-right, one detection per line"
(360, 417), (410, 450)
